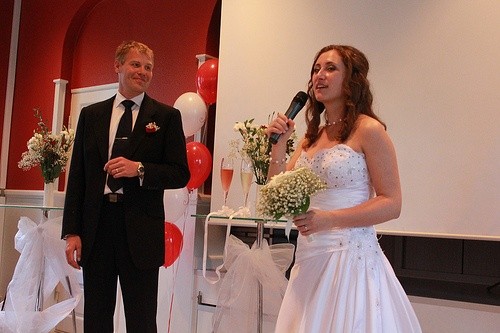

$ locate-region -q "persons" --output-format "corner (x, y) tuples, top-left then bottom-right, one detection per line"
(263, 45), (425, 333)
(61, 38), (190, 333)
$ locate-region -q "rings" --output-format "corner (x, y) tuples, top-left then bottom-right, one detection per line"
(116, 169), (119, 172)
(305, 226), (308, 230)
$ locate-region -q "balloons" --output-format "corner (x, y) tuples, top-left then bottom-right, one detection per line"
(163, 58), (220, 268)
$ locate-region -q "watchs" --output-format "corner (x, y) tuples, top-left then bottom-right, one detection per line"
(137, 162), (144, 177)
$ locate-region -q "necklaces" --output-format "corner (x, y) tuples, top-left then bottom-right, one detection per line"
(327, 117), (347, 126)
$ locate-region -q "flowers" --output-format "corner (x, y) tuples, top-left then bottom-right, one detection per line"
(146, 121), (161, 133)
(230, 111), (299, 186)
(16, 106), (76, 184)
(255, 167), (328, 224)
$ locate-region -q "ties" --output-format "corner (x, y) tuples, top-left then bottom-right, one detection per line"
(107, 100), (136, 193)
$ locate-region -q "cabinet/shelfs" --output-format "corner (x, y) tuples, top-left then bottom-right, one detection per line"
(190, 215), (500, 333)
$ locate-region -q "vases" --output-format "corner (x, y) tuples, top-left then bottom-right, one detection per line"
(43, 182), (54, 207)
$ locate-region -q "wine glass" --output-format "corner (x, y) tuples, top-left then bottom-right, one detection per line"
(241, 158), (254, 207)
(221, 157), (234, 211)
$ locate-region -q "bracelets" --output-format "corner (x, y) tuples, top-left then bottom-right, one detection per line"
(271, 158), (286, 164)
